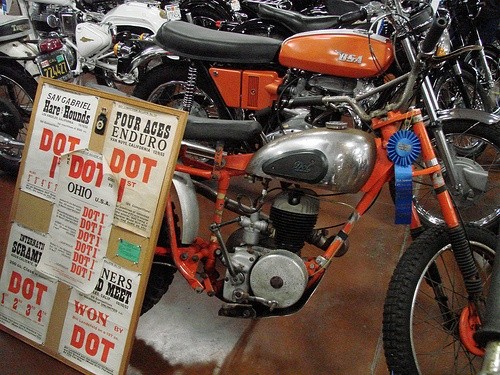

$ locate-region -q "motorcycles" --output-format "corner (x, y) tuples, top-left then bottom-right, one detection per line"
(0, 0), (500, 375)
